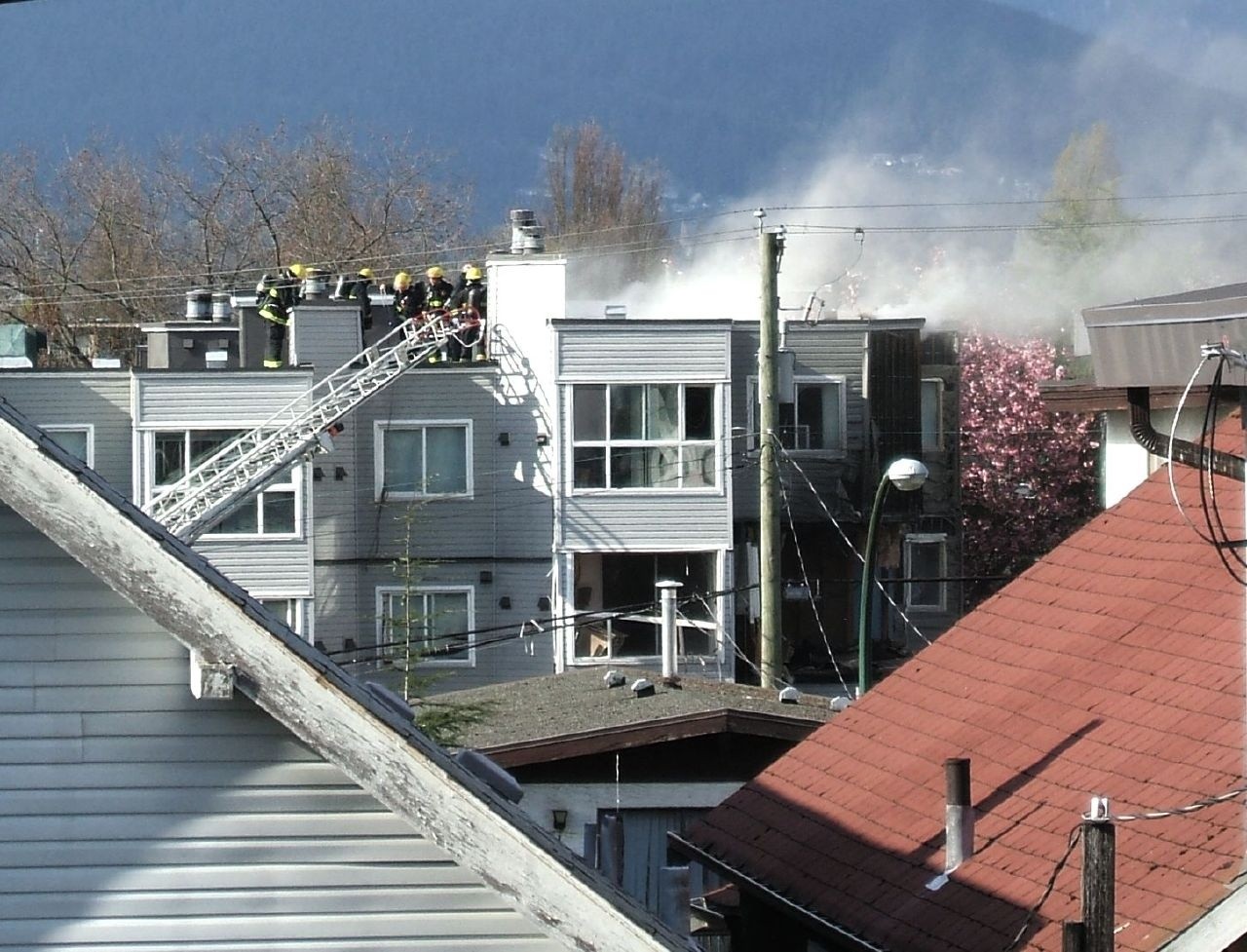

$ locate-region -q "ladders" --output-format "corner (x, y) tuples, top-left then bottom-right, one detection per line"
(144, 306), (482, 545)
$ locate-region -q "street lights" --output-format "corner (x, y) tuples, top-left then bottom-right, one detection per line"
(854, 458), (928, 692)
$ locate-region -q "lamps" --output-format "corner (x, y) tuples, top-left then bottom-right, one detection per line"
(552, 809), (568, 830)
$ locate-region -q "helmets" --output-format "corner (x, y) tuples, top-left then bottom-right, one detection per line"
(465, 268), (483, 280)
(289, 264), (305, 281)
(462, 264), (476, 273)
(357, 269), (375, 285)
(426, 266), (443, 279)
(393, 272), (412, 290)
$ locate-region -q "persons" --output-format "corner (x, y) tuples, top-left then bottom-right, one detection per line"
(254, 264), (377, 367)
(380, 264), (486, 364)
